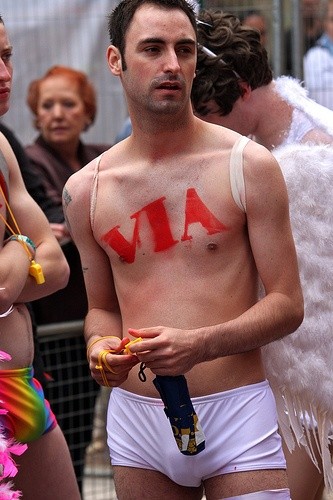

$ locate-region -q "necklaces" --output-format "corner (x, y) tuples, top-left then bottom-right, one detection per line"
(0, 188), (45, 285)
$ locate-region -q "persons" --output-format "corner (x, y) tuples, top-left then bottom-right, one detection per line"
(0, 17), (81, 500)
(234, 10), (267, 49)
(22, 65), (114, 500)
(299, 0), (333, 112)
(62, 0), (304, 500)
(190, 9), (333, 500)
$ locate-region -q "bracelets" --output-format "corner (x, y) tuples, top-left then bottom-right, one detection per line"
(11, 235), (35, 249)
